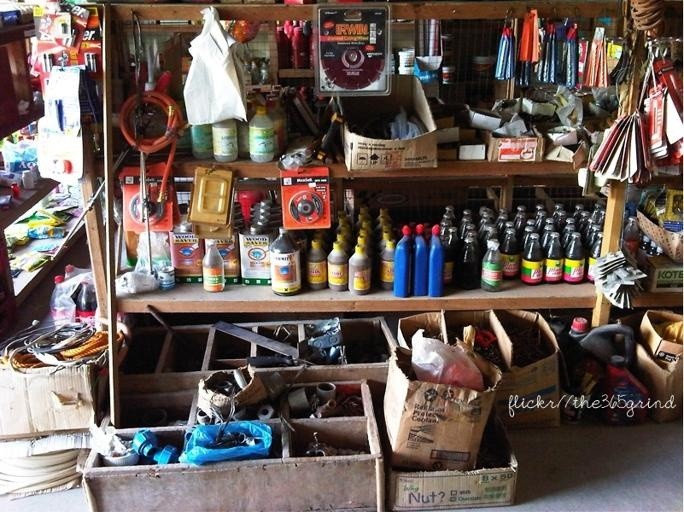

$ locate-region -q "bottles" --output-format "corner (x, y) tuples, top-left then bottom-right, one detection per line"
(201, 204), (664, 299)
(190, 97), (289, 163)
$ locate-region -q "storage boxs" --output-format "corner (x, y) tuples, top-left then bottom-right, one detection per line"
(638, 251), (683, 294)
(340, 75), (439, 172)
(0, 310), (683, 512)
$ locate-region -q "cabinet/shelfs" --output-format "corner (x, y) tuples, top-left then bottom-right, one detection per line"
(83, 1), (683, 329)
(0, 178), (85, 307)
